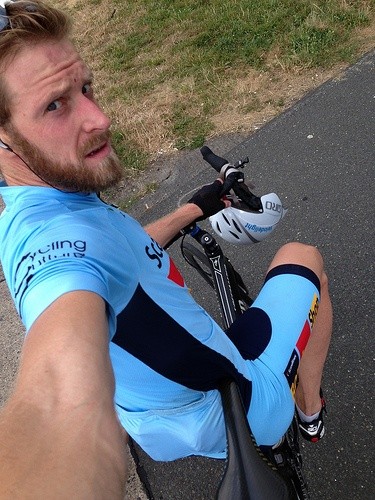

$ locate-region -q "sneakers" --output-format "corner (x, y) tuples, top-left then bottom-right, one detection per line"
(293, 374), (326, 443)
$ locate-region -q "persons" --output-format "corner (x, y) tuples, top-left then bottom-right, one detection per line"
(1, 0), (334, 500)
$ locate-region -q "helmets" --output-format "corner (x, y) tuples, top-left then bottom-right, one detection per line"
(208, 191), (286, 246)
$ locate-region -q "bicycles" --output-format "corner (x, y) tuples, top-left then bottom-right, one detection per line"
(179, 147), (309, 499)
